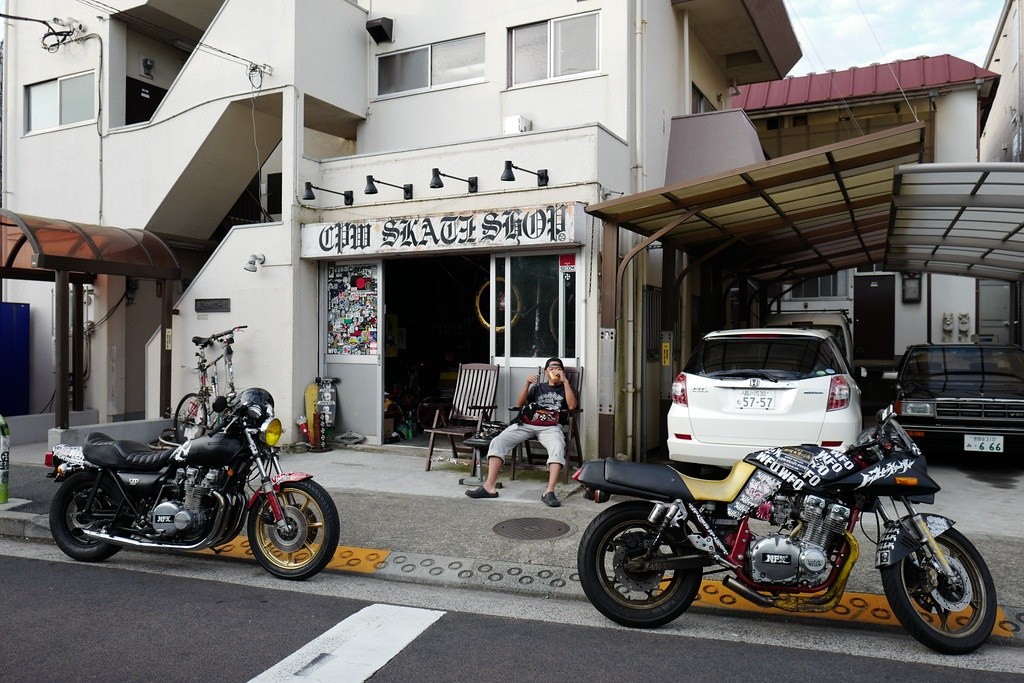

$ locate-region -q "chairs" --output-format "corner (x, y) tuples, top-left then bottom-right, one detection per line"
(969, 362), (997, 370)
(508, 366), (584, 485)
(424, 362), (501, 477)
(915, 362), (942, 373)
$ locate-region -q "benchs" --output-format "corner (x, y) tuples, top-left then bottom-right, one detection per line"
(459, 434), (503, 488)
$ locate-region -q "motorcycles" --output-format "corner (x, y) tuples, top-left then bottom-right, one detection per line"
(42, 389), (340, 580)
(568, 391), (996, 656)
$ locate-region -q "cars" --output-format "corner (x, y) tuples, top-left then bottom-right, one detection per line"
(666, 323), (863, 476)
(880, 344), (1023, 466)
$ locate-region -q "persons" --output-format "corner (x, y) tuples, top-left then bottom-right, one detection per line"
(465, 357), (577, 507)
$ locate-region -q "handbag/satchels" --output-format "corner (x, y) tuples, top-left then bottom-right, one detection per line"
(522, 405), (559, 425)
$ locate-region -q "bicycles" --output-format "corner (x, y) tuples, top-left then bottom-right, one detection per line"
(173, 324), (248, 446)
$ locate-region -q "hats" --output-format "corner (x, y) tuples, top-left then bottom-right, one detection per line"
(543, 357), (565, 371)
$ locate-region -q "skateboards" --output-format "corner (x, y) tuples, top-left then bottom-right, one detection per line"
(304, 382), (319, 444)
(314, 376), (340, 428)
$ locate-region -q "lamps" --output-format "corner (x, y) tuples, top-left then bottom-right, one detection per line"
(500, 160), (549, 186)
(244, 254), (265, 272)
(365, 175), (413, 200)
(429, 168), (478, 193)
(366, 17), (393, 44)
(302, 182), (354, 206)
(174, 39), (195, 53)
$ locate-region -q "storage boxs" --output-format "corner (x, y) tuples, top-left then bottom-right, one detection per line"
(384, 418), (394, 439)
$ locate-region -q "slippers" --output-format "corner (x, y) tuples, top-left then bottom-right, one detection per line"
(464, 485), (499, 498)
(541, 491), (562, 507)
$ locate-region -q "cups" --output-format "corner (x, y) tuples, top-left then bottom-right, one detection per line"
(548, 372), (558, 381)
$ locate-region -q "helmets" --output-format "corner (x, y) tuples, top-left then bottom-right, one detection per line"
(235, 387), (274, 419)
